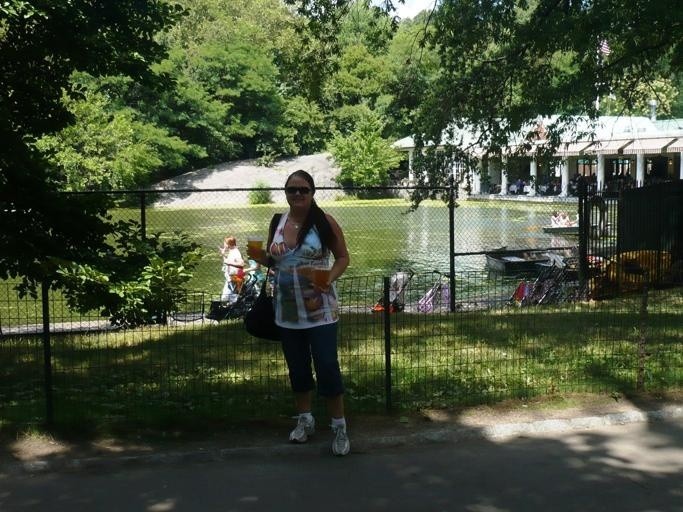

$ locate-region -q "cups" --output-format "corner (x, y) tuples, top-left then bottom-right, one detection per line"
(310, 264), (333, 293)
(247, 234), (265, 260)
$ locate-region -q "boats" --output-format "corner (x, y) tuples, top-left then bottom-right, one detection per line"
(599, 248), (670, 286)
(538, 221), (613, 238)
(484, 246), (551, 277)
(545, 235), (605, 276)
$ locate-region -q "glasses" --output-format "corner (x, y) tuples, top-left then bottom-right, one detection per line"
(286, 187), (310, 194)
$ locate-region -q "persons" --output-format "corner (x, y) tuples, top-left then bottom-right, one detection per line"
(514, 172), (629, 193)
(242, 256), (264, 296)
(550, 208), (579, 227)
(267, 267), (275, 295)
(219, 235), (244, 303)
(244, 170), (349, 458)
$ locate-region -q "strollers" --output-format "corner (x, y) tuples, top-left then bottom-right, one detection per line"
(416, 269), (450, 313)
(504, 264), (592, 307)
(366, 267), (415, 313)
(206, 273), (259, 322)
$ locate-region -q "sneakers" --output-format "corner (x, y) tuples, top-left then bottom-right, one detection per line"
(289, 413), (315, 443)
(329, 422), (350, 456)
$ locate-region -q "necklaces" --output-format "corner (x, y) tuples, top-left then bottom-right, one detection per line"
(288, 215), (302, 232)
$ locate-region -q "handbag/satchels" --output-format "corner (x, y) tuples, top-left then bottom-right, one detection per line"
(245, 292), (285, 340)
